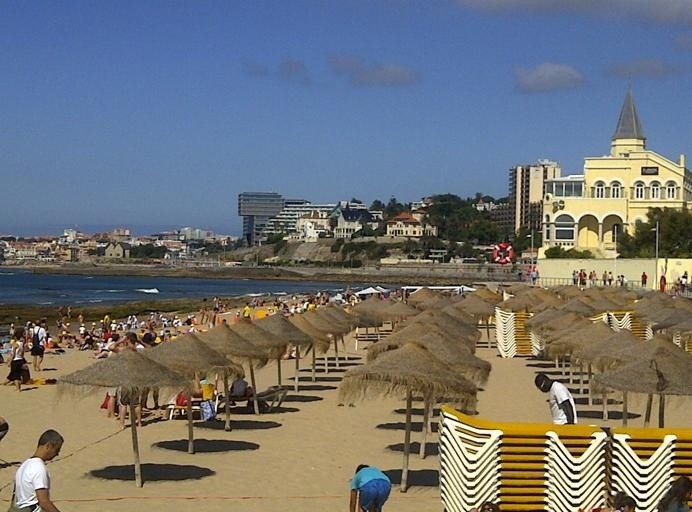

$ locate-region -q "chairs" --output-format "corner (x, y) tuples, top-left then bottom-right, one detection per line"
(166, 395), (221, 420)
(217, 390), (287, 412)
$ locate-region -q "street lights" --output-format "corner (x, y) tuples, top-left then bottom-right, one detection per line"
(613, 222), (629, 288)
(651, 221), (659, 290)
(526, 228), (534, 286)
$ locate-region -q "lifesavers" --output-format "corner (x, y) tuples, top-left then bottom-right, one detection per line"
(0, 338), (12, 353)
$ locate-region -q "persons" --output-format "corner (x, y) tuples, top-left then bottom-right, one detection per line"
(0, 417), (10, 441)
(1, 291), (356, 428)
(469, 501), (499, 511)
(520, 266), (688, 297)
(349, 464), (392, 512)
(612, 476), (691, 512)
(10, 428), (64, 512)
